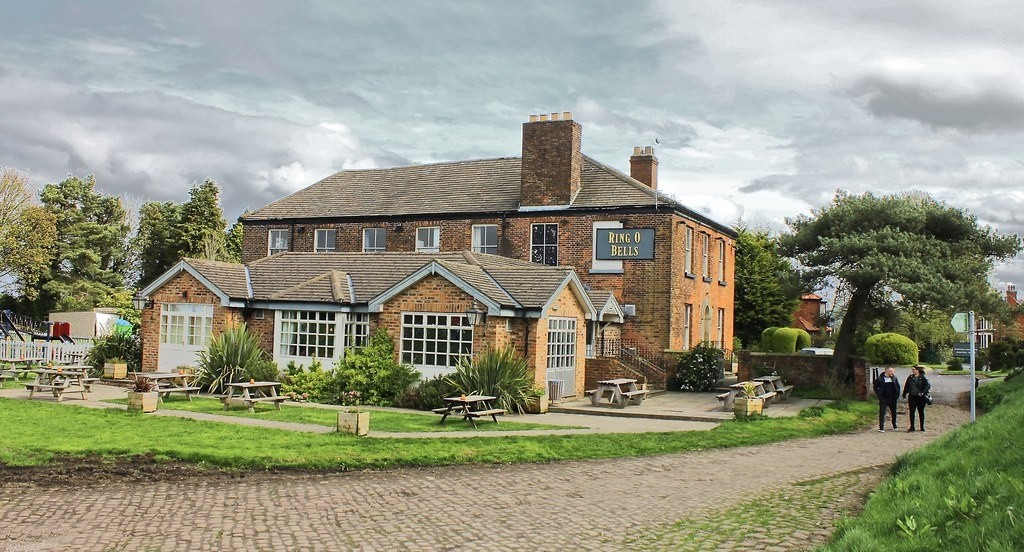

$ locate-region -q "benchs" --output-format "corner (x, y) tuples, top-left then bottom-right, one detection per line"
(0, 367), (101, 389)
(431, 406), (471, 411)
(153, 387), (201, 392)
(716, 385), (794, 399)
(214, 394), (291, 402)
(585, 387), (649, 396)
(468, 409), (508, 415)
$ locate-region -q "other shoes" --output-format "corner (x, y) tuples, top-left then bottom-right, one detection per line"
(892, 426), (898, 431)
(907, 427), (916, 432)
(920, 427), (925, 431)
(878, 427), (884, 433)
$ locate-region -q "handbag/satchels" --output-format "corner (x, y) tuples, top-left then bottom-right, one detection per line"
(923, 394), (933, 405)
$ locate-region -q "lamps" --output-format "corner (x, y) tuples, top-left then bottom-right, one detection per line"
(464, 297), (487, 325)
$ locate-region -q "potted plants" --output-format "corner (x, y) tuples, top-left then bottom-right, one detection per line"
(734, 384), (763, 417)
(337, 403), (369, 437)
(121, 375), (158, 412)
(103, 356), (127, 380)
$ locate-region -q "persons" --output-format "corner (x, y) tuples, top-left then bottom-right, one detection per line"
(873, 366), (901, 432)
(902, 366), (930, 431)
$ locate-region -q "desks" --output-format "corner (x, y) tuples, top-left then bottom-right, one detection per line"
(753, 376), (793, 404)
(137, 373), (198, 404)
(724, 381), (775, 412)
(435, 395), (504, 429)
(220, 381), (285, 414)
(591, 379), (642, 408)
(0, 357), (93, 402)
